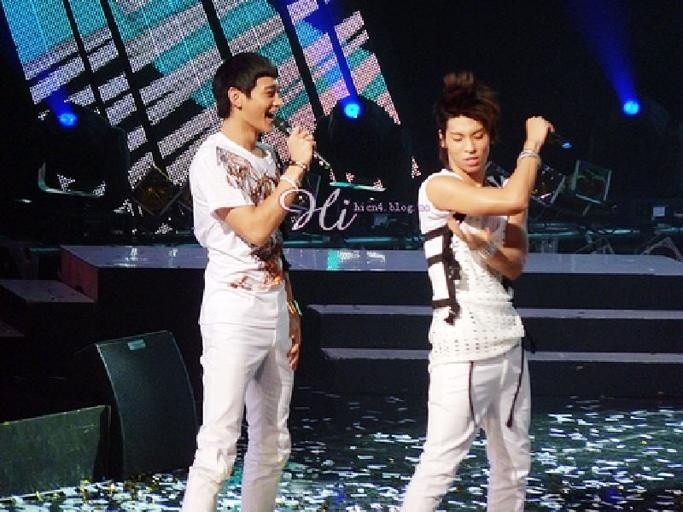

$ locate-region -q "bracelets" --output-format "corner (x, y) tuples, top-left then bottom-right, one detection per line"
(279, 174), (301, 195)
(286, 159), (308, 176)
(517, 149), (543, 170)
(477, 239), (498, 260)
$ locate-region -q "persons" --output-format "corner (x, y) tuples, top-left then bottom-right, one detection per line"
(399, 70), (555, 512)
(180, 51), (318, 512)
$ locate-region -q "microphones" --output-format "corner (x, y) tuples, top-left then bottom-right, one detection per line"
(273, 118), (331, 170)
